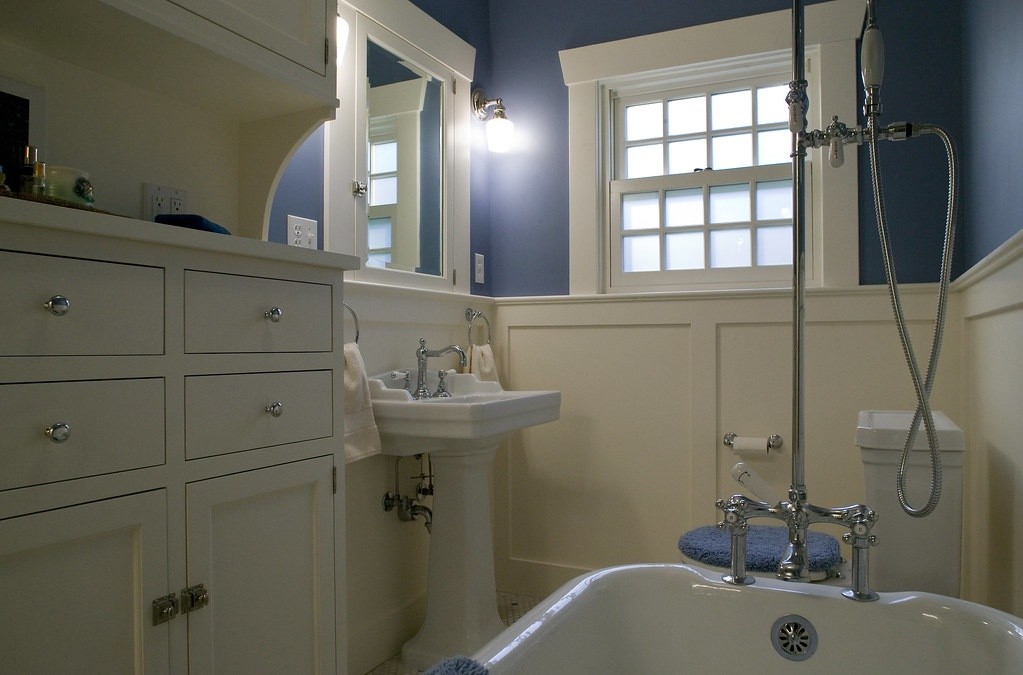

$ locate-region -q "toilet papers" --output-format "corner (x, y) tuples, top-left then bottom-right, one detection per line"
(732, 436), (770, 456)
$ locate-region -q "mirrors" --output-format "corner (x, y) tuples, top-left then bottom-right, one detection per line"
(366, 33), (445, 278)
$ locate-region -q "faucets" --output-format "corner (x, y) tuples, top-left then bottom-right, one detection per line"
(413, 338), (468, 401)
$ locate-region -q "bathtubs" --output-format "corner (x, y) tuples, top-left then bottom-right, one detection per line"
(468, 563), (1023, 675)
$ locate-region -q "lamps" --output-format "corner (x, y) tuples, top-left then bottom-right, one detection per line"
(471, 87), (514, 152)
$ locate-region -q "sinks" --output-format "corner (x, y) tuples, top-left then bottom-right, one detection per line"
(367, 369), (562, 458)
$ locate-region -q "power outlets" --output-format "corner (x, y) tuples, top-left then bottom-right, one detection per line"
(146, 185), (187, 223)
(287, 214), (317, 251)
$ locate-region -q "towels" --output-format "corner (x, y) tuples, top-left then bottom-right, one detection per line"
(423, 656), (490, 675)
(343, 341), (383, 466)
(462, 344), (499, 383)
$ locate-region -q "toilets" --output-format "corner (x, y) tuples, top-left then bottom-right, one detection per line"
(677, 409), (967, 599)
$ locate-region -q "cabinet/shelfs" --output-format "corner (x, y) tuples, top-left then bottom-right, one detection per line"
(0, 196), (359, 674)
